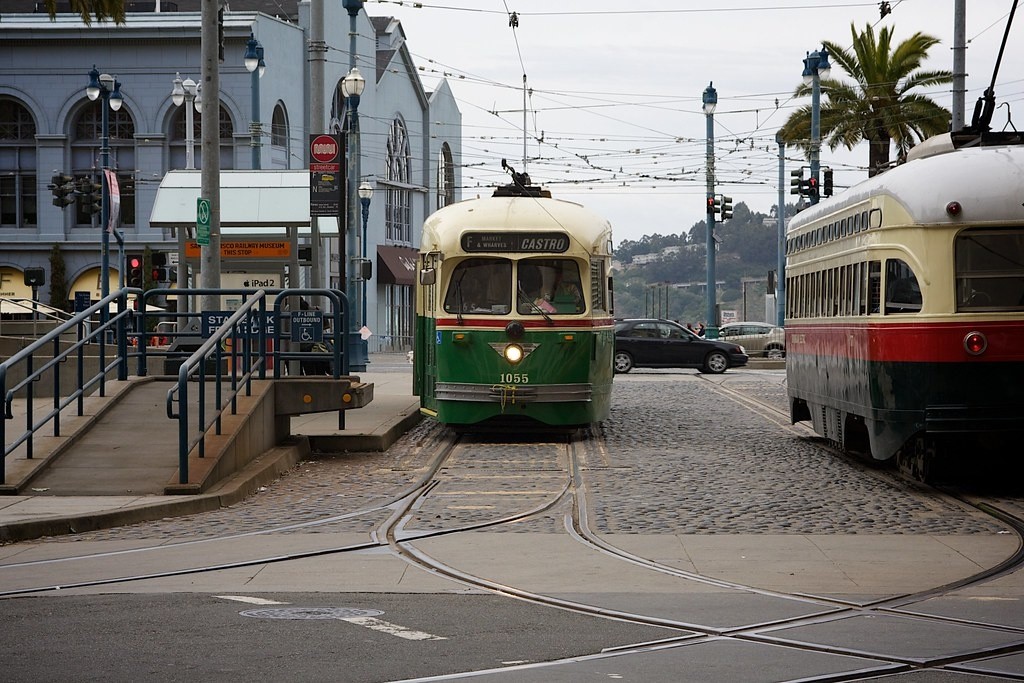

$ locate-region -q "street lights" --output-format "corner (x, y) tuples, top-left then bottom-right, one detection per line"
(356, 177), (375, 365)
(85, 63), (125, 343)
(170, 71), (202, 170)
(243, 32), (266, 170)
(802, 45), (834, 207)
(700, 79), (718, 328)
(339, 67), (371, 373)
(775, 122), (788, 327)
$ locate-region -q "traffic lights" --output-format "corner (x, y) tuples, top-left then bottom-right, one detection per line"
(62, 172), (76, 208)
(51, 172), (62, 207)
(706, 196), (721, 214)
(126, 254), (143, 294)
(808, 177), (818, 197)
(790, 169), (802, 194)
(150, 253), (167, 281)
(824, 170), (834, 196)
(722, 195), (734, 221)
(86, 178), (103, 214)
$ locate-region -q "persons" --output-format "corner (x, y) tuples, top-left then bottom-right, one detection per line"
(670, 319), (682, 339)
(686, 323), (697, 334)
(150, 328), (167, 346)
(697, 322), (705, 337)
(528, 268), (580, 305)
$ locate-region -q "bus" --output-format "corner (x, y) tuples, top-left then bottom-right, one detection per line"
(782, 123), (1024, 489)
(412, 159), (615, 434)
(155, 321), (177, 347)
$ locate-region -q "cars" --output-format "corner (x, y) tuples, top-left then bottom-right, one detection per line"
(700, 321), (785, 360)
(614, 318), (749, 375)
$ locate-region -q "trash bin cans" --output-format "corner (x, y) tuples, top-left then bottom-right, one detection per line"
(301, 340), (336, 376)
(164, 317), (230, 375)
